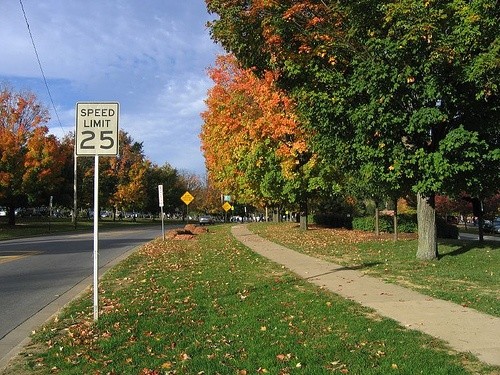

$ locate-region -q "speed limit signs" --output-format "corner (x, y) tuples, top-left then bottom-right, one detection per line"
(75, 102), (119, 156)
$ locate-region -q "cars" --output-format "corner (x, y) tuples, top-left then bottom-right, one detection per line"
(199, 216), (215, 226)
(0, 206), (138, 220)
